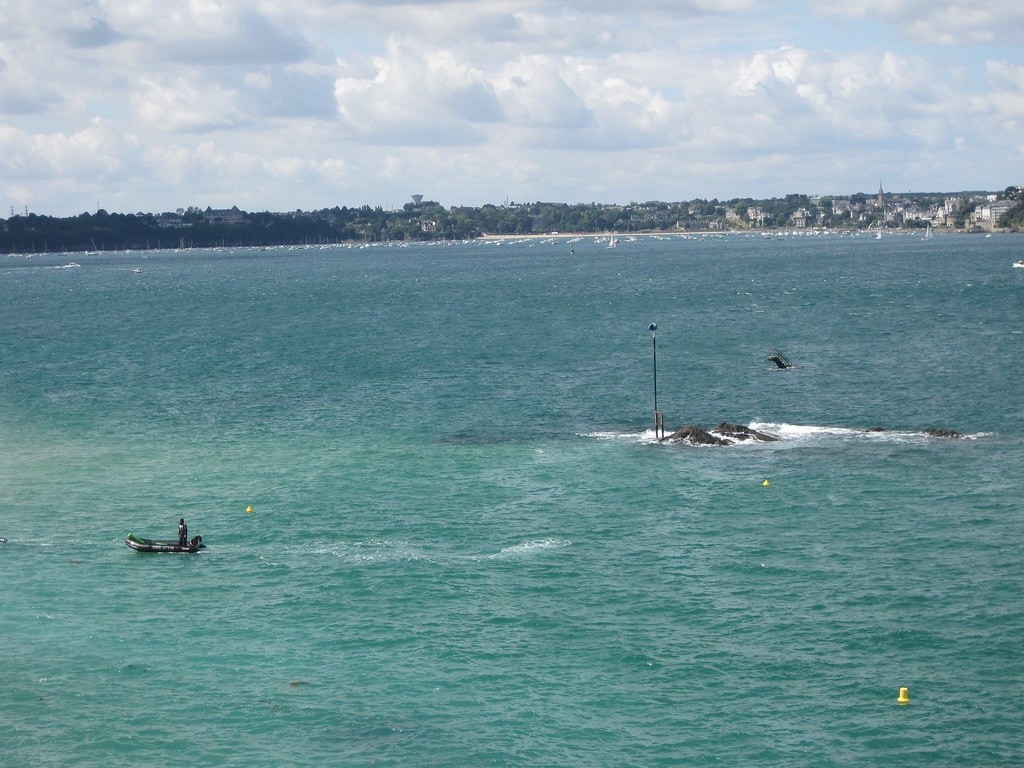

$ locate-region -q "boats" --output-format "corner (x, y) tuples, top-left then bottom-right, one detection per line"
(123, 533), (208, 553)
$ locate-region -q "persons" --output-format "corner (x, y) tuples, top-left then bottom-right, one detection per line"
(177, 517), (188, 548)
